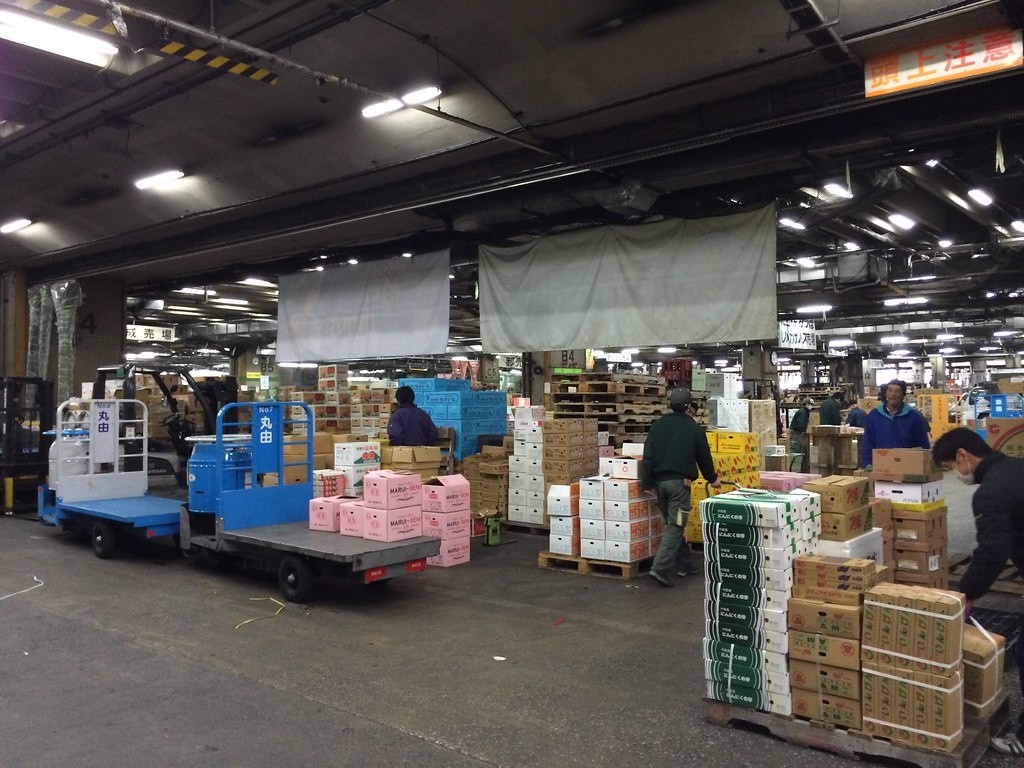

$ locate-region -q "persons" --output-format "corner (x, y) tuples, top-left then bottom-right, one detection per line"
(820, 392), (844, 425)
(933, 426), (1024, 617)
(789, 397), (816, 454)
(862, 379), (931, 467)
(642, 387), (722, 587)
(845, 404), (867, 427)
(387, 386), (439, 447)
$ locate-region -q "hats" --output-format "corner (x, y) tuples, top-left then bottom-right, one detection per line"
(887, 379), (907, 396)
(690, 403), (699, 411)
(802, 397), (816, 406)
(670, 387), (694, 405)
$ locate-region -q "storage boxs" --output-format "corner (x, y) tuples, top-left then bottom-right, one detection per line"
(114, 363), (1024, 745)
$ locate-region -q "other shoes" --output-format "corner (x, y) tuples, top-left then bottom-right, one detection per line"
(677, 566), (700, 576)
(649, 568), (674, 586)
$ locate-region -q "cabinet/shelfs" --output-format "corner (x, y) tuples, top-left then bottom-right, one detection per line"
(548, 372), (666, 449)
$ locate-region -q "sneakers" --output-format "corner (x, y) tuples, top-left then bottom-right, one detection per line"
(989, 732), (1024, 756)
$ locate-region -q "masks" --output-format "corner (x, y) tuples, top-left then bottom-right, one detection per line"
(953, 459), (974, 486)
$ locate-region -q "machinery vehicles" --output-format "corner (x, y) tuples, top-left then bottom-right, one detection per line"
(0, 376), (53, 521)
(736, 377), (784, 439)
(92, 362), (219, 491)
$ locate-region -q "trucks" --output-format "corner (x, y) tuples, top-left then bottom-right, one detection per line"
(961, 386), (1024, 429)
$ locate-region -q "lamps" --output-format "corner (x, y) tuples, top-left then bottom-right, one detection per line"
(0, 3), (118, 71)
(361, 83), (442, 118)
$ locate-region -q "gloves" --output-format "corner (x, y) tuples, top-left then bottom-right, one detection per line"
(966, 597), (973, 620)
